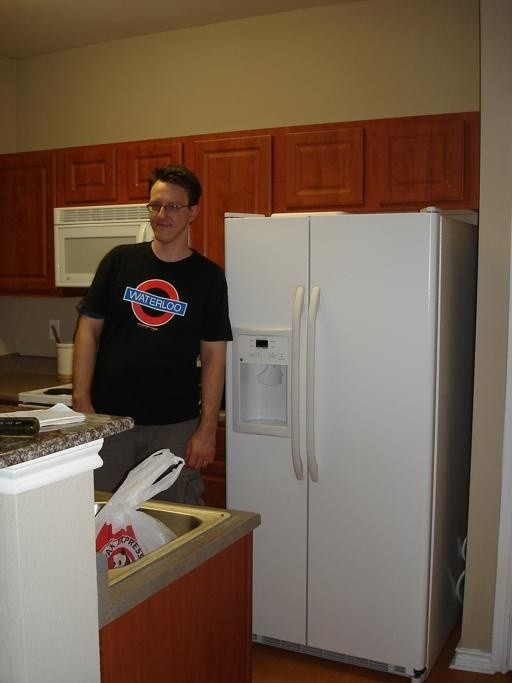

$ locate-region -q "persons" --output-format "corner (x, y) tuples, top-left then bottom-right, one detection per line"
(71, 164), (233, 505)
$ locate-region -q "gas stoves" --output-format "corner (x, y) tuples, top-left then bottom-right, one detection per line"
(19, 384), (72, 407)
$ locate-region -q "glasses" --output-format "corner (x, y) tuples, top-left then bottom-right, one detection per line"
(146, 202), (189, 213)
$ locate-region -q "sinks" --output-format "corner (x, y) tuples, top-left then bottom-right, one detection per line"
(95, 497), (231, 587)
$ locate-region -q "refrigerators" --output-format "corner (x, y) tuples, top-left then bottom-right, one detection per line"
(222, 208), (476, 683)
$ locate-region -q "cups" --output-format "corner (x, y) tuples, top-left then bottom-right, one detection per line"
(56, 342), (75, 384)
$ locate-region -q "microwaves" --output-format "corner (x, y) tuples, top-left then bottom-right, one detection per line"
(53, 203), (160, 289)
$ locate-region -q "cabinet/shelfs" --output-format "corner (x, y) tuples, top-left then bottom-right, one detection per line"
(0, 150), (59, 300)
(274, 107), (482, 215)
(183, 126), (274, 271)
(58, 136), (183, 206)
